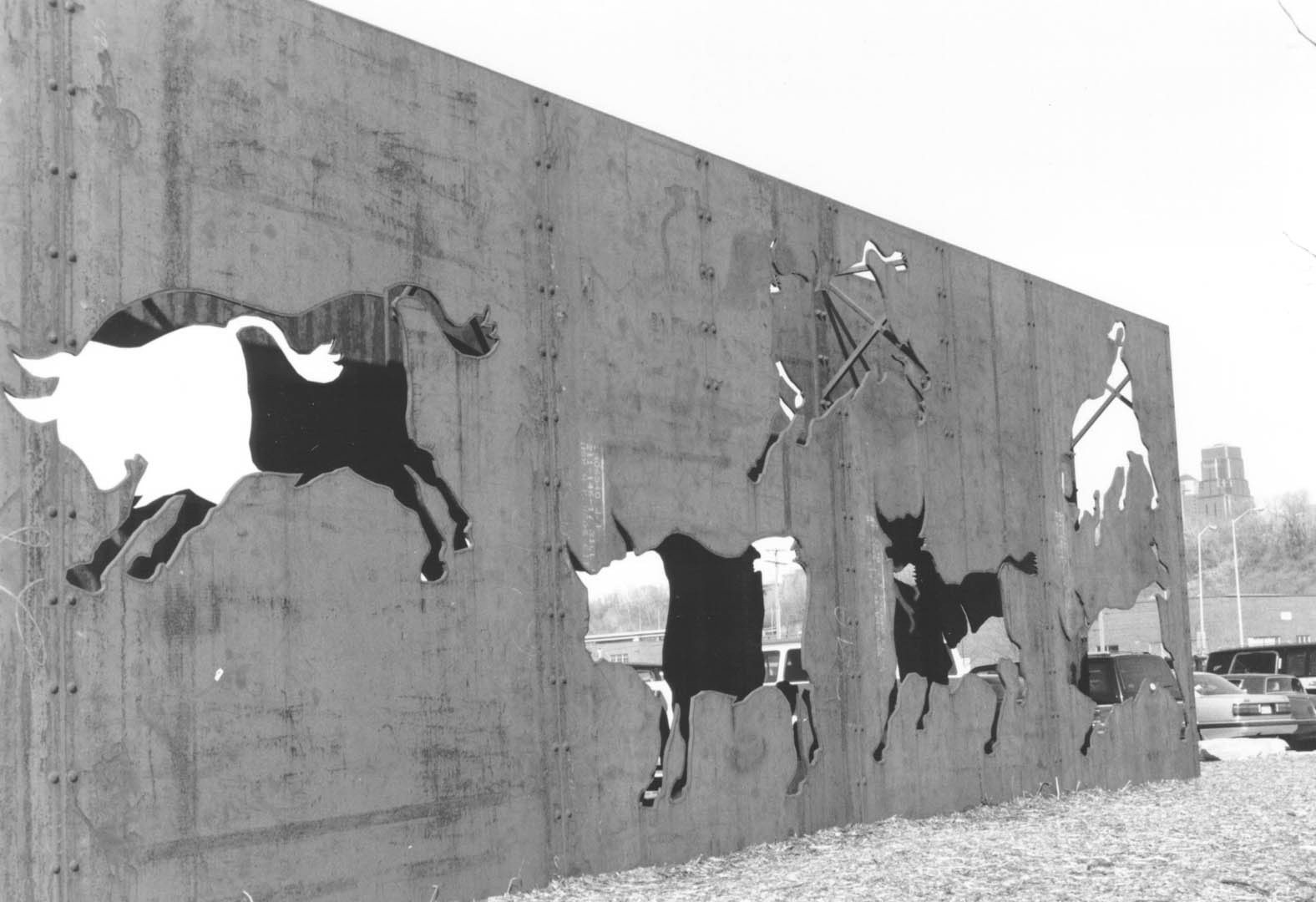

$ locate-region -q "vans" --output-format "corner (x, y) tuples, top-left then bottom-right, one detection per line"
(968, 645), (1183, 751)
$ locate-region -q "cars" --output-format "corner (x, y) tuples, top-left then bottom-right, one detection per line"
(1168, 670), (1316, 741)
